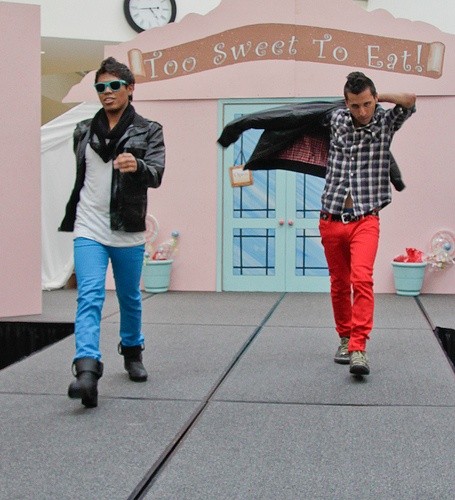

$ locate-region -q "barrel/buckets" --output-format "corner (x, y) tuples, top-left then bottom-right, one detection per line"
(392, 261), (428, 297)
(143, 260), (172, 294)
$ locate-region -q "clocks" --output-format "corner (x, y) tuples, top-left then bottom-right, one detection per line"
(123, 0), (177, 33)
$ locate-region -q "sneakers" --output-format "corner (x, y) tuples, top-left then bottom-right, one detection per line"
(349, 350), (372, 376)
(334, 337), (351, 362)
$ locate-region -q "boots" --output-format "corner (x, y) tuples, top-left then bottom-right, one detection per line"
(118, 341), (147, 383)
(68, 357), (103, 407)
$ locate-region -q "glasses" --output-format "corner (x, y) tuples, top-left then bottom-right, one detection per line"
(95, 80), (128, 94)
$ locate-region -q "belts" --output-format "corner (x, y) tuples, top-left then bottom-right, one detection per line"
(319, 210), (379, 223)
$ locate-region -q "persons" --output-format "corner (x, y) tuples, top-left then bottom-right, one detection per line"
(58, 57), (165, 408)
(217, 71), (416, 377)
